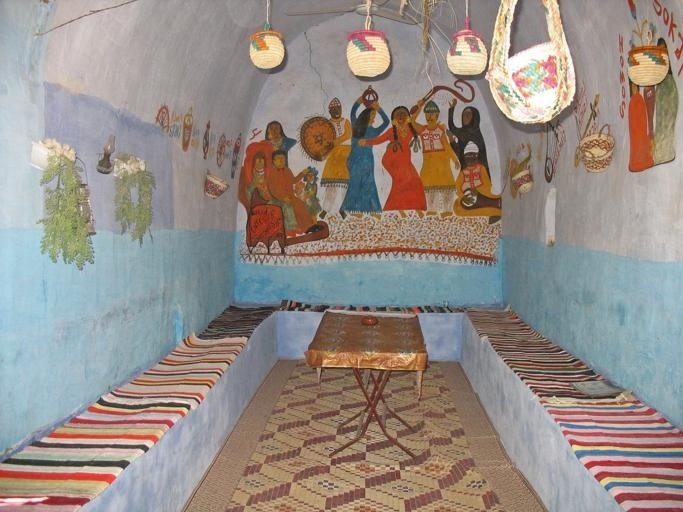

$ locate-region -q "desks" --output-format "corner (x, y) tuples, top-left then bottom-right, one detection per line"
(303, 311), (428, 459)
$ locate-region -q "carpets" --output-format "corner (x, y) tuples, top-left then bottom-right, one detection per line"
(223, 359), (505, 512)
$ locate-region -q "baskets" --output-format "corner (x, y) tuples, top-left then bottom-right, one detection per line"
(627, 46), (670, 87)
(249, 30), (285, 69)
(205, 174), (229, 199)
(446, 29), (488, 76)
(511, 170), (533, 194)
(579, 125), (615, 173)
(347, 29), (391, 78)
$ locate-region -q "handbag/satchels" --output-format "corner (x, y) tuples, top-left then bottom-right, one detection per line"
(485, 0), (576, 124)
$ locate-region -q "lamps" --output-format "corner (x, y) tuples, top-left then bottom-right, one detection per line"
(97, 134), (116, 175)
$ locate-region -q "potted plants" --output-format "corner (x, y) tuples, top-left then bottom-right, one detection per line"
(29, 138), (96, 271)
(113, 154), (157, 248)
